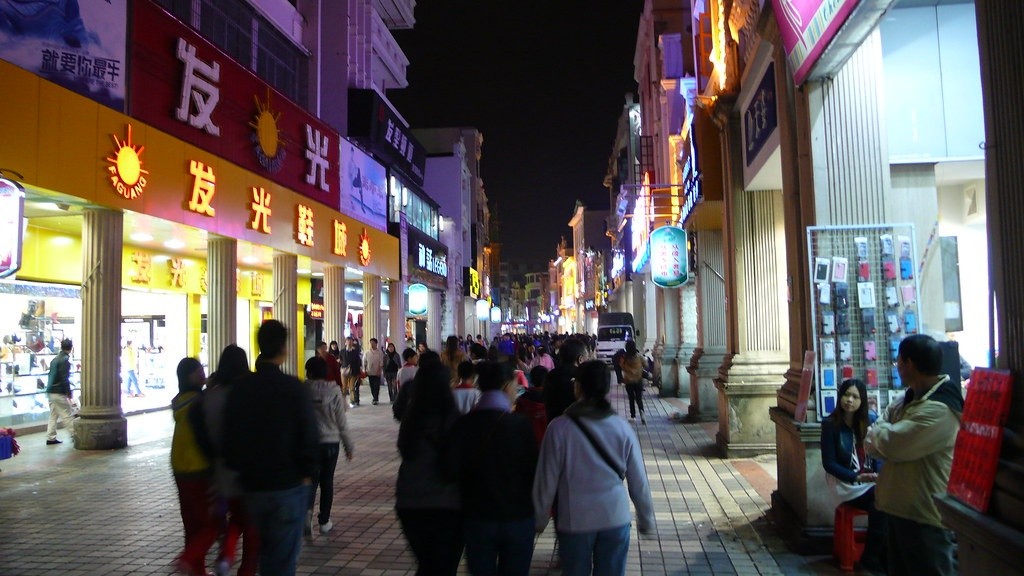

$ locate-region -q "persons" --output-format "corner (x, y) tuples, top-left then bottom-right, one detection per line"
(392, 351), (441, 422)
(170, 358), (224, 575)
(457, 328), (600, 387)
(618, 339), (649, 425)
(821, 378), (888, 576)
(439, 335), (470, 390)
(395, 348), (419, 395)
(864, 334), (965, 576)
(611, 348), (626, 385)
(224, 320), (322, 575)
(434, 358), (540, 576)
(304, 355), (353, 534)
(316, 341), (343, 393)
(46, 339), (77, 445)
(383, 343), (401, 405)
(511, 365), (549, 451)
(327, 336), (362, 408)
(451, 361), (483, 415)
(540, 338), (590, 427)
(394, 359), (468, 576)
(364, 338), (385, 405)
(418, 342), (430, 360)
(532, 359), (655, 576)
(187, 343), (259, 575)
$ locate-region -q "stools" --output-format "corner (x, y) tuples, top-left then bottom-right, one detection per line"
(832, 502), (871, 573)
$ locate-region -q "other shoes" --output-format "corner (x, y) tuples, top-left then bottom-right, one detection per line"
(47, 439), (63, 444)
(321, 521), (335, 532)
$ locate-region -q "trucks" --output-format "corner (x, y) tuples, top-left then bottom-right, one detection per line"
(593, 312), (640, 368)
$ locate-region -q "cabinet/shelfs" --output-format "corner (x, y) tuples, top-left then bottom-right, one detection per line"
(0, 324), (80, 398)
